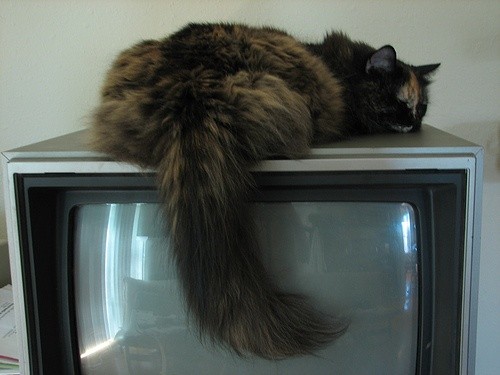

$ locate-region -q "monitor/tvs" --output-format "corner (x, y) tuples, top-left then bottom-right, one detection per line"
(3, 124), (485, 375)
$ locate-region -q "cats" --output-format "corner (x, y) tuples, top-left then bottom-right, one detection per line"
(78, 21), (442, 362)
